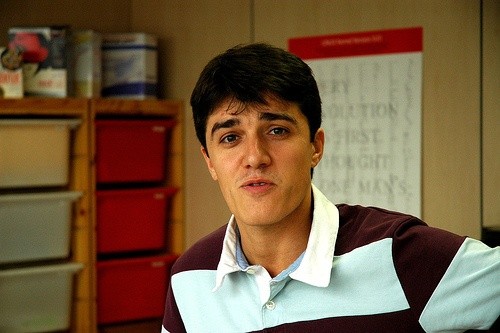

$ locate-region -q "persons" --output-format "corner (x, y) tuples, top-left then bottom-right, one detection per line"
(160, 44), (500, 333)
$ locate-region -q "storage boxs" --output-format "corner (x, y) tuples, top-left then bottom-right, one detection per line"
(0, 27), (180, 333)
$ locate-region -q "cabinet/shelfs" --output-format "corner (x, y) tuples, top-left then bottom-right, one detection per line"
(0, 98), (187, 333)
